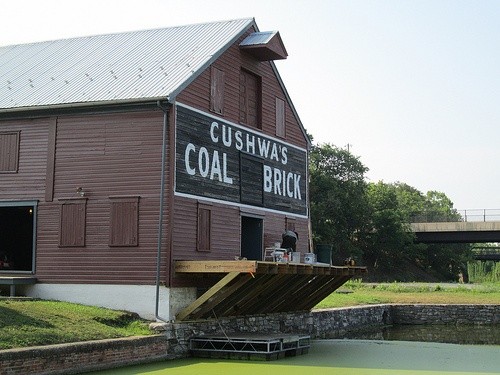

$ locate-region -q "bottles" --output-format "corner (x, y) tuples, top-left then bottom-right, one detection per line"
(282, 252), (289, 263)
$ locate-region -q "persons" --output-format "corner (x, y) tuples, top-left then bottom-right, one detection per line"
(280, 230), (299, 253)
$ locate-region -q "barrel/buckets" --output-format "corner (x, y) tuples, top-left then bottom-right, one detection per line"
(304, 253), (316, 264)
(271, 251), (284, 262)
